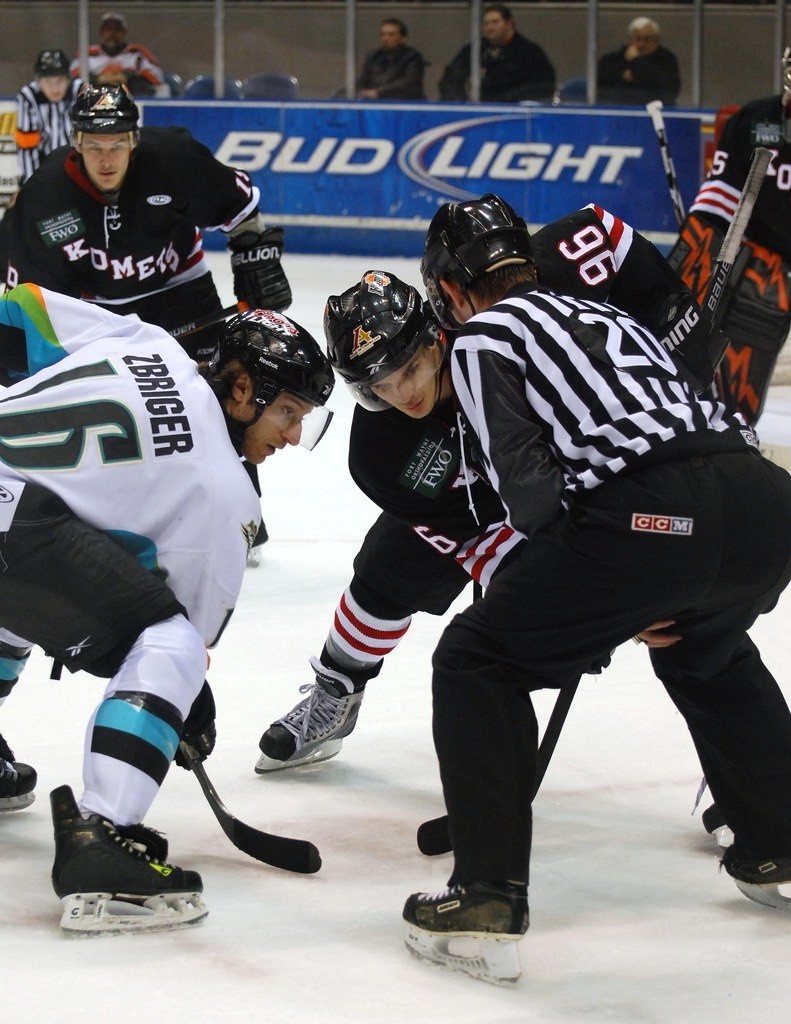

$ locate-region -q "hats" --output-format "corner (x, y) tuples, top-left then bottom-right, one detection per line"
(100, 13), (126, 32)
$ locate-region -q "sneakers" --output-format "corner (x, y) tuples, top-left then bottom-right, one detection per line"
(400, 860), (531, 989)
(721, 834), (791, 912)
(49, 786), (208, 935)
(254, 659), (384, 774)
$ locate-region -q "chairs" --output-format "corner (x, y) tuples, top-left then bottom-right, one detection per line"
(160, 73), (300, 102)
(553, 78), (590, 104)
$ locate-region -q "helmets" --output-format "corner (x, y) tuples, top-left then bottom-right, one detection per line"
(420, 193), (535, 305)
(70, 84), (136, 134)
(325, 270), (429, 391)
(35, 50), (69, 78)
(212, 311), (336, 409)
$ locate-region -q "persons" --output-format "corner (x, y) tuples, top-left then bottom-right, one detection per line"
(0, 84), (293, 566)
(671, 43), (791, 423)
(358, 19), (428, 101)
(253, 203), (715, 775)
(595, 15), (683, 107)
(66, 12), (165, 103)
(9, 49), (95, 213)
(400, 194), (791, 985)
(0, 281), (334, 938)
(438, 4), (556, 104)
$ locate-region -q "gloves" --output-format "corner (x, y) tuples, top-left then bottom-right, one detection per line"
(652, 299), (728, 397)
(224, 226), (292, 314)
(172, 681), (216, 770)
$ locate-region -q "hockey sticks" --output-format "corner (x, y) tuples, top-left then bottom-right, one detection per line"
(702, 148), (773, 314)
(191, 765), (327, 876)
(169, 300), (251, 336)
(418, 680), (582, 860)
(645, 100), (686, 225)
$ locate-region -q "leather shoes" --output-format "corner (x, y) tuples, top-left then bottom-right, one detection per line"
(0, 753), (36, 811)
